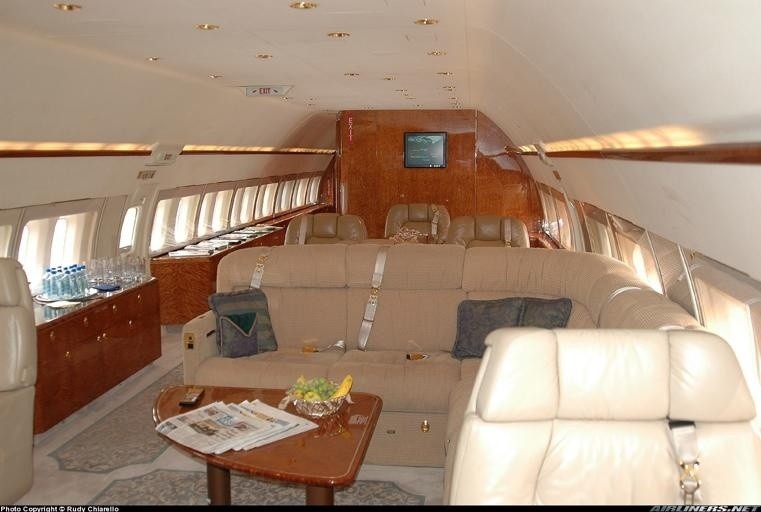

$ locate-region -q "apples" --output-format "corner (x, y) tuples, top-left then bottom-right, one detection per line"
(292, 388), (303, 398)
(304, 392), (320, 402)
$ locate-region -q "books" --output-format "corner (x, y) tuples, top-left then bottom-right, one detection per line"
(167, 222), (278, 257)
(153, 395), (320, 455)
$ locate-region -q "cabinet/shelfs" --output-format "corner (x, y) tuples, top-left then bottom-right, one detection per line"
(33, 228), (284, 434)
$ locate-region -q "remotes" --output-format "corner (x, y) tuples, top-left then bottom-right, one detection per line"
(179, 388), (205, 407)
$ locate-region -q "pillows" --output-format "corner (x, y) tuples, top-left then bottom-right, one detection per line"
(451, 296), (573, 360)
(207, 287), (279, 360)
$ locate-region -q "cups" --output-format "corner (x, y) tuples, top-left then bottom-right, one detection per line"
(87, 254), (145, 287)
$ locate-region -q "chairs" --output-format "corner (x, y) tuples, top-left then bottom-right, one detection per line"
(283, 201), (530, 248)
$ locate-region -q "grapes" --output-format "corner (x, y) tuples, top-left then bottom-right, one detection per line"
(291, 376), (333, 400)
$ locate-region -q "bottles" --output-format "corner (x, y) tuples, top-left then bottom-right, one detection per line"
(42, 263), (90, 300)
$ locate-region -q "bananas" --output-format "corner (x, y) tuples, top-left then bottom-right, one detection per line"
(327, 374), (353, 401)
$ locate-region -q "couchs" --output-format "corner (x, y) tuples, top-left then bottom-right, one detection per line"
(179, 247), (760, 509)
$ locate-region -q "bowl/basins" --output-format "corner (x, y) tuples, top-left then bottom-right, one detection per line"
(288, 388), (347, 419)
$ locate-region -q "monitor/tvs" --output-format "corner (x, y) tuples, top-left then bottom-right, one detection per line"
(403, 131), (448, 168)
